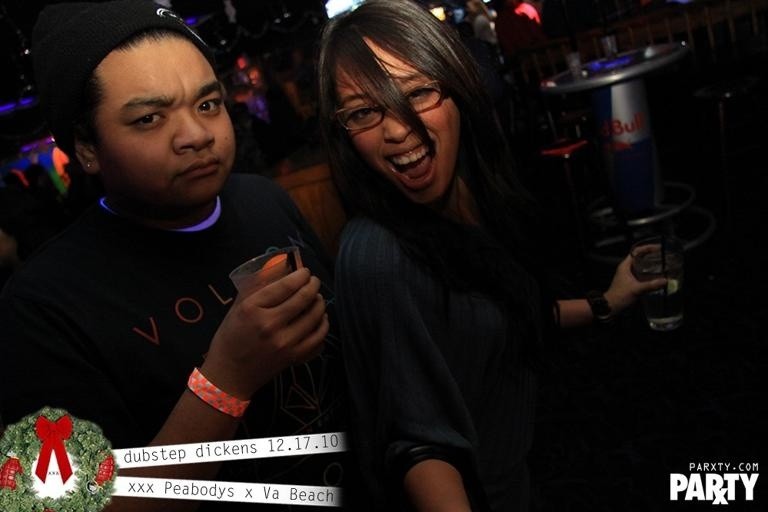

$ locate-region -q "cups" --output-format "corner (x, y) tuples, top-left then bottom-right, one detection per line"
(601, 35), (619, 61)
(229, 243), (324, 374)
(564, 51), (583, 74)
(629, 236), (694, 334)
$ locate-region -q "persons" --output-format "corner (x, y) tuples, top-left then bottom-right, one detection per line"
(0, 0), (554, 283)
(316, 0), (673, 512)
(0, 0), (339, 512)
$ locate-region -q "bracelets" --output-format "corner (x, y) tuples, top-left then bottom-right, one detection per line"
(185, 368), (251, 419)
(586, 293), (611, 324)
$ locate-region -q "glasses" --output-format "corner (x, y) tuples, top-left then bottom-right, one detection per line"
(334, 79), (442, 131)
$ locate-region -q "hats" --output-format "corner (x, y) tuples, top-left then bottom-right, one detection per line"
(24, 2), (216, 158)
